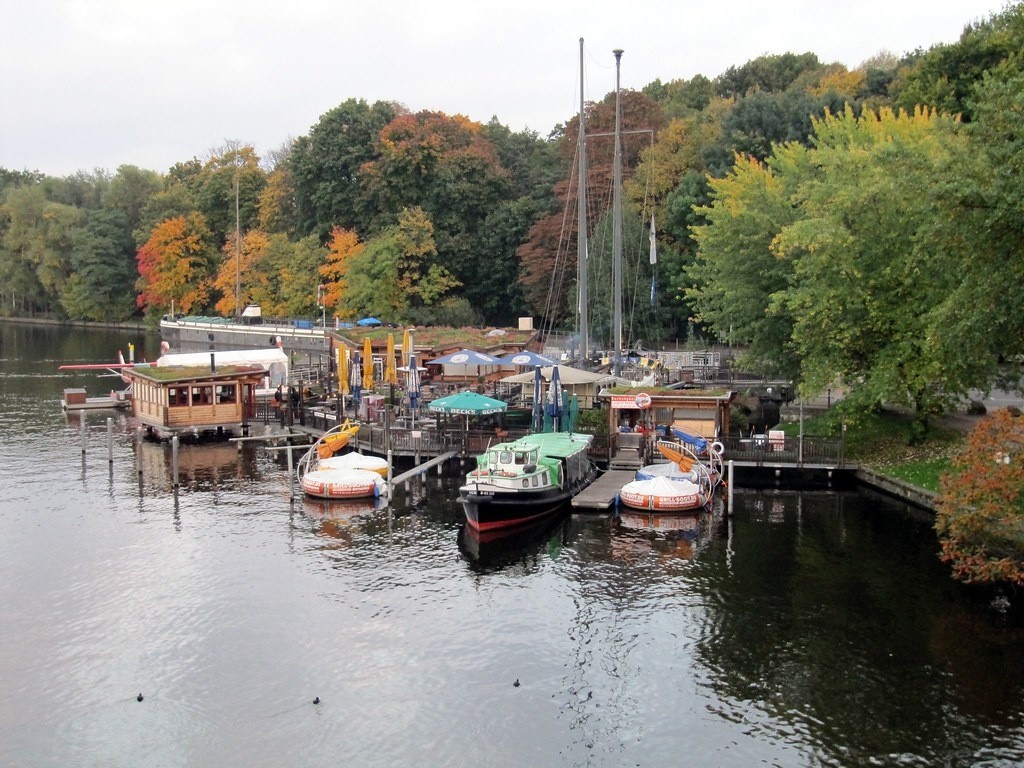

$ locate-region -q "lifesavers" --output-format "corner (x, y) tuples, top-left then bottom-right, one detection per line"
(636, 392), (651, 409)
(499, 471), (517, 476)
(470, 470), (488, 475)
(711, 442), (725, 455)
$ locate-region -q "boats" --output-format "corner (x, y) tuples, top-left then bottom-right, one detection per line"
(58, 341), (172, 409)
(619, 426), (729, 514)
(301, 469), (385, 500)
(316, 451), (390, 479)
(455, 426), (601, 550)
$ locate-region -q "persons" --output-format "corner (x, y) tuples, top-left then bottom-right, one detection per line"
(275, 385), (314, 420)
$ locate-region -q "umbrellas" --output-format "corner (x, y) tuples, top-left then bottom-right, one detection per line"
(363, 337), (373, 393)
(384, 333), (398, 383)
(316, 435), (349, 460)
(560, 389), (569, 432)
(406, 356), (421, 429)
(426, 390), (508, 430)
(338, 342), (350, 414)
(543, 391), (553, 432)
(568, 393), (579, 431)
(350, 351), (361, 404)
(491, 349), (554, 373)
(669, 427), (708, 453)
(655, 443), (698, 476)
(401, 329), (410, 386)
(357, 317), (382, 327)
(545, 365), (564, 432)
(326, 425), (360, 445)
(424, 350), (501, 387)
(531, 365), (542, 433)
